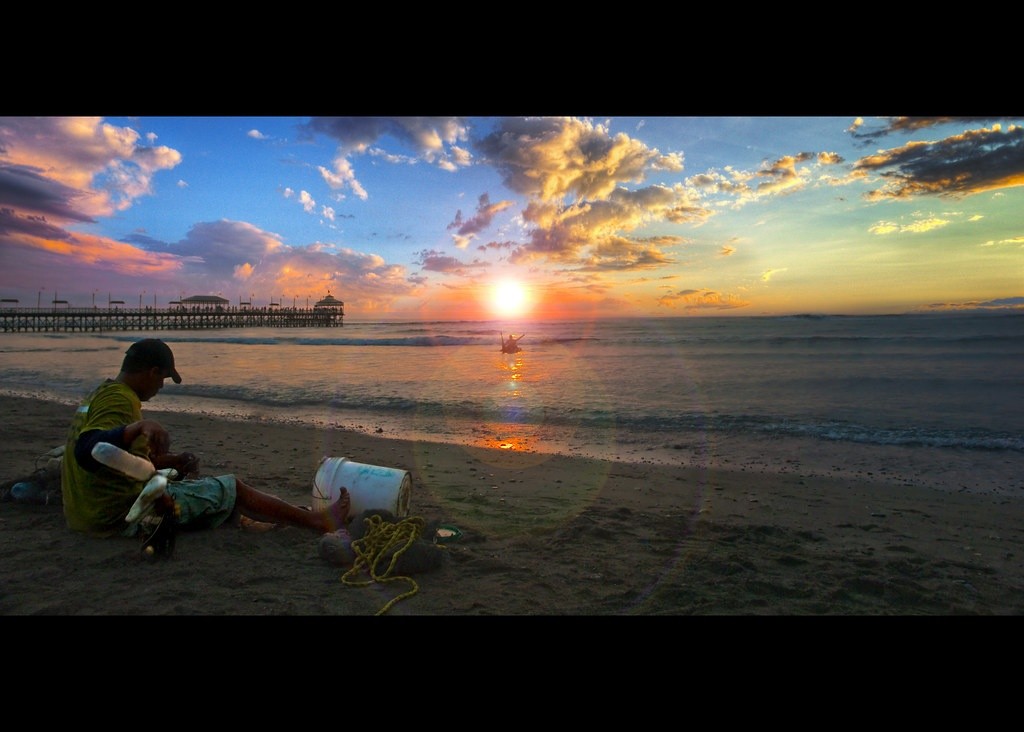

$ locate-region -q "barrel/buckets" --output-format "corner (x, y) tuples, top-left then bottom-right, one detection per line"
(312, 458), (412, 520)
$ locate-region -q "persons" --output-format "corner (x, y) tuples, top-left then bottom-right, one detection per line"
(61, 339), (351, 539)
(504, 333), (517, 348)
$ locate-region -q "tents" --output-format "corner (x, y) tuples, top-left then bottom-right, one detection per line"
(316, 296), (344, 309)
(181, 296), (230, 310)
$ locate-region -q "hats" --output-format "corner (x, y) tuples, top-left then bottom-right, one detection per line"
(126, 337), (182, 384)
